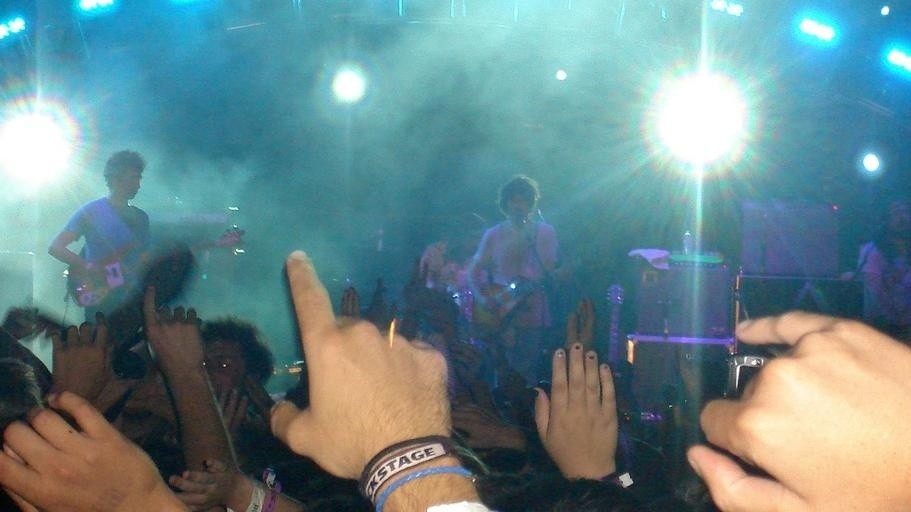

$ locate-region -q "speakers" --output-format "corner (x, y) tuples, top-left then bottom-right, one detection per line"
(631, 338), (731, 410)
(635, 253), (734, 339)
(741, 201), (841, 278)
(735, 276), (863, 358)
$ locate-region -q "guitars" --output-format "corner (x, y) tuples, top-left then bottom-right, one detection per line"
(601, 283), (642, 435)
(66, 225), (246, 308)
(473, 250), (590, 336)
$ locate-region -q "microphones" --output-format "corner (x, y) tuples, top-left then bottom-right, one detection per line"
(515, 208), (527, 224)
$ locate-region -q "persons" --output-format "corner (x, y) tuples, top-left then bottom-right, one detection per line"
(47, 150), (216, 339)
(418, 207), (486, 332)
(468, 171), (564, 382)
(1, 250), (911, 512)
(854, 195), (911, 351)
